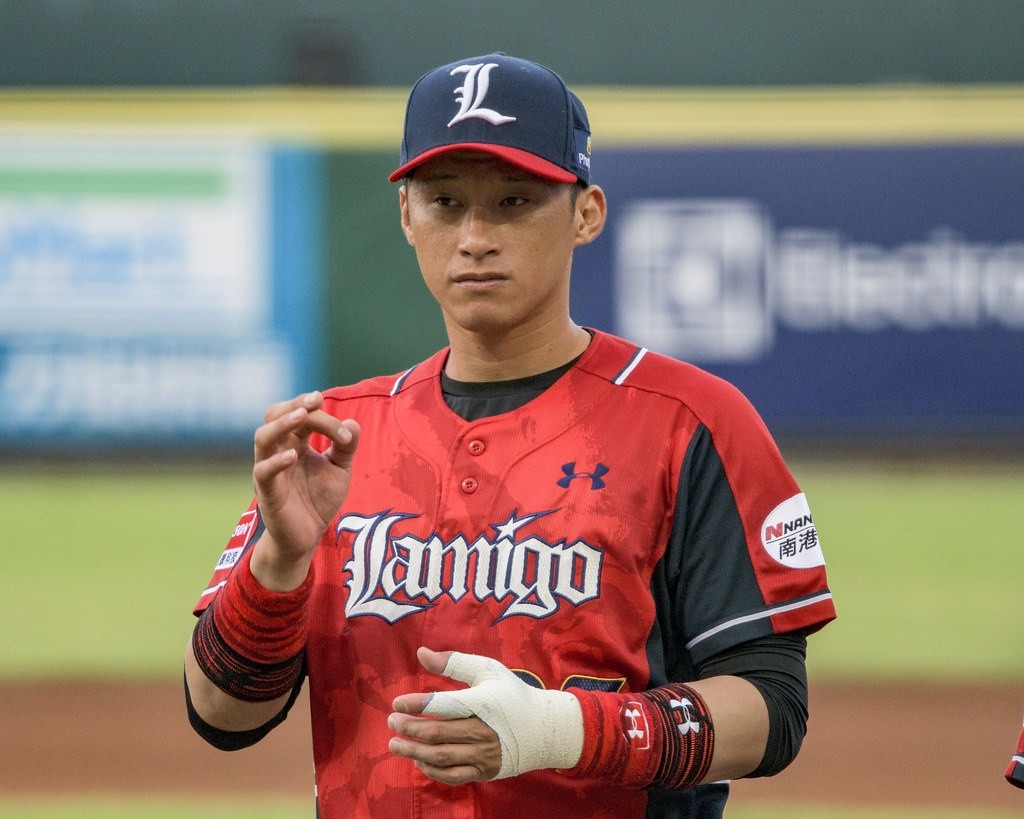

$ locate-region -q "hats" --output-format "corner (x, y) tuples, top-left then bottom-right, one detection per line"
(388, 51), (592, 187)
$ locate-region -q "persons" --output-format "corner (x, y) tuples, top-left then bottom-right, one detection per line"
(181, 51), (836, 819)
